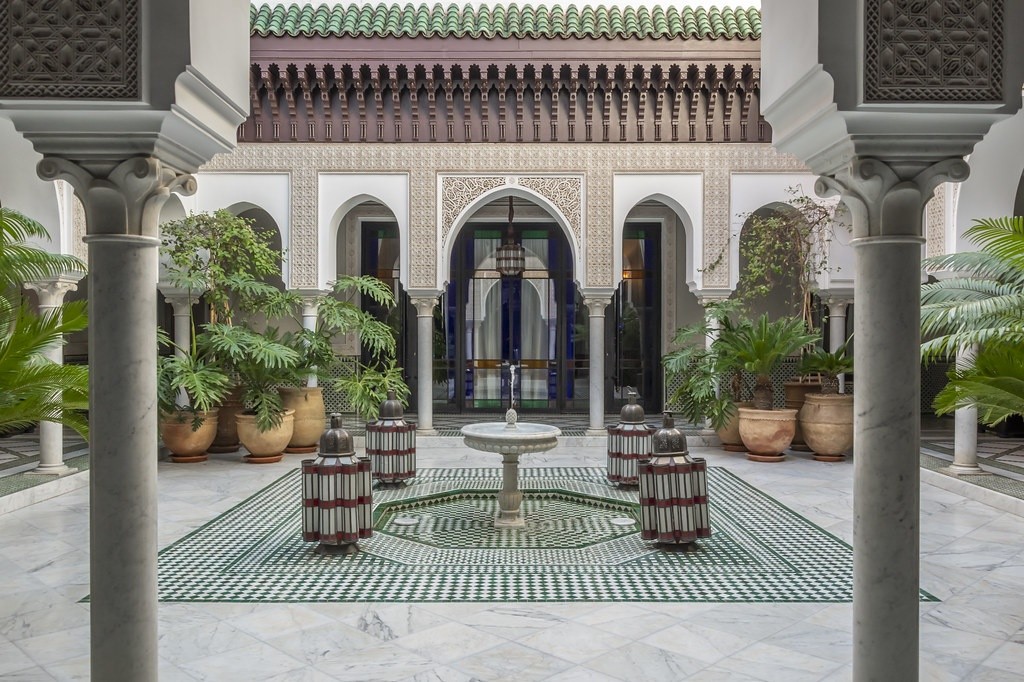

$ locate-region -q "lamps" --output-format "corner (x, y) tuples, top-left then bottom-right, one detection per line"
(495, 195), (526, 276)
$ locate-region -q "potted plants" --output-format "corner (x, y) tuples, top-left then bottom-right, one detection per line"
(662, 183), (853, 464)
(159, 206), (412, 463)
